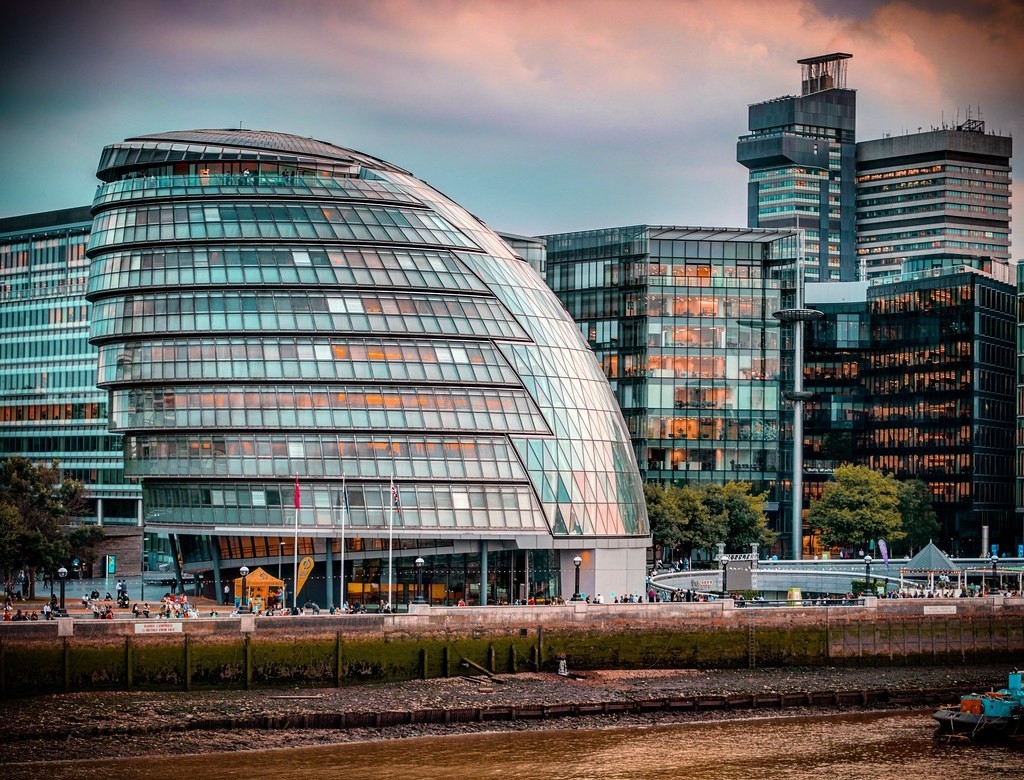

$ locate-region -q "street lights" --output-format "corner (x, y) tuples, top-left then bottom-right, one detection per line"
(278, 541), (286, 583)
(141, 537), (149, 601)
(413, 558), (424, 604)
(991, 553), (998, 593)
(863, 553), (873, 596)
(53, 568), (69, 618)
(719, 556), (730, 599)
(571, 556), (584, 602)
(238, 565), (249, 616)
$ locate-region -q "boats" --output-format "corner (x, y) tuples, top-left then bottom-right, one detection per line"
(931, 668), (1024, 744)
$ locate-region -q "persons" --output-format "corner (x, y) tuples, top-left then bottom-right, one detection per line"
(96, 168), (296, 188)
(457, 598), (465, 607)
(766, 548), (1012, 565)
(0, 563), (130, 621)
(496, 588), (715, 605)
(646, 557), (689, 593)
(730, 593), (765, 608)
(376, 599), (413, 614)
(132, 577), (368, 618)
(807, 573), (1024, 606)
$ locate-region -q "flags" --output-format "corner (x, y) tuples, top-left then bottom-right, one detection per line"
(344, 484), (350, 514)
(392, 481), (399, 513)
(294, 471), (301, 509)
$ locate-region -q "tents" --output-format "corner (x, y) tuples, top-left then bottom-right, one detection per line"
(900, 539), (961, 575)
